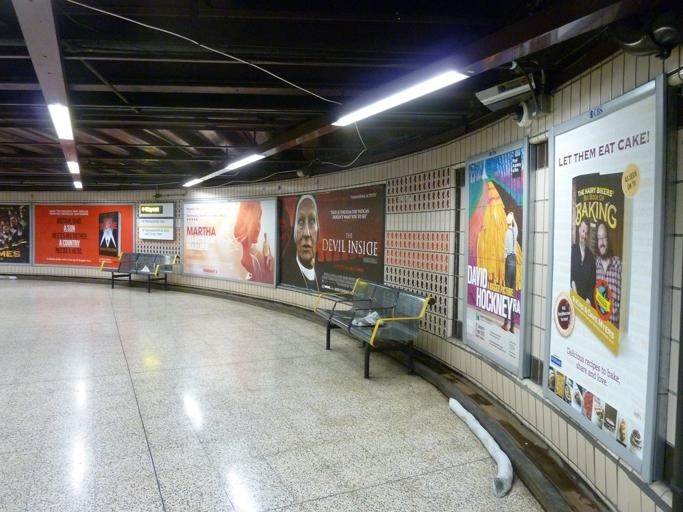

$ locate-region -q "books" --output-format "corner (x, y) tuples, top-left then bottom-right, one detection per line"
(350, 311), (383, 326)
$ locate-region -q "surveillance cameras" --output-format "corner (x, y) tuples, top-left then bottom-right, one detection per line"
(473, 72), (536, 113)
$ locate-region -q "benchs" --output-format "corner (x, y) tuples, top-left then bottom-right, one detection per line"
(311, 277), (434, 381)
(98, 252), (180, 293)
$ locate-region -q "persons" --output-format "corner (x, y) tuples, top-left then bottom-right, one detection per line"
(8, 215), (26, 241)
(569, 215), (596, 308)
(0, 218), (8, 242)
(499, 210), (521, 334)
(232, 199), (273, 285)
(593, 219), (623, 329)
(280, 194), (337, 293)
